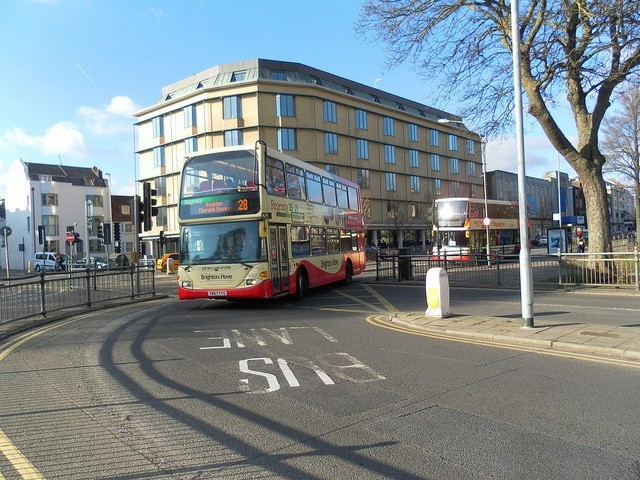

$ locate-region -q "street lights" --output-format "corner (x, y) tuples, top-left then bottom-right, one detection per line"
(437, 118), (491, 267)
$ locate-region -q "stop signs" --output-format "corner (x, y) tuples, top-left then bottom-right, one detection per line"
(67, 233), (75, 242)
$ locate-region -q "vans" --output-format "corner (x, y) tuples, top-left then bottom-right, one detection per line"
(34, 252), (70, 271)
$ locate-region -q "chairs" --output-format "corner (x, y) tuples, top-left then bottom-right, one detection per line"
(213, 179), (225, 194)
(469, 207), (479, 218)
(199, 181), (212, 197)
(246, 181), (257, 192)
(226, 180), (238, 193)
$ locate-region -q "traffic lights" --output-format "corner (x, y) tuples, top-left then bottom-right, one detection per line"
(136, 195), (143, 233)
(143, 183), (158, 231)
(160, 231), (164, 248)
(98, 226), (102, 238)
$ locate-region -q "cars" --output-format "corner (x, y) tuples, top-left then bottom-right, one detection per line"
(83, 257), (107, 269)
(539, 236), (547, 246)
(531, 238), (539, 246)
(366, 245), (387, 261)
(157, 253), (178, 272)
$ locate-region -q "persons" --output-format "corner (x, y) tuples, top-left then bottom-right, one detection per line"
(53, 253), (66, 272)
(376, 240), (386, 259)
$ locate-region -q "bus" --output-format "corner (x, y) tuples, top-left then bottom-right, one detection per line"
(431, 197), (530, 263)
(177, 139), (366, 301)
